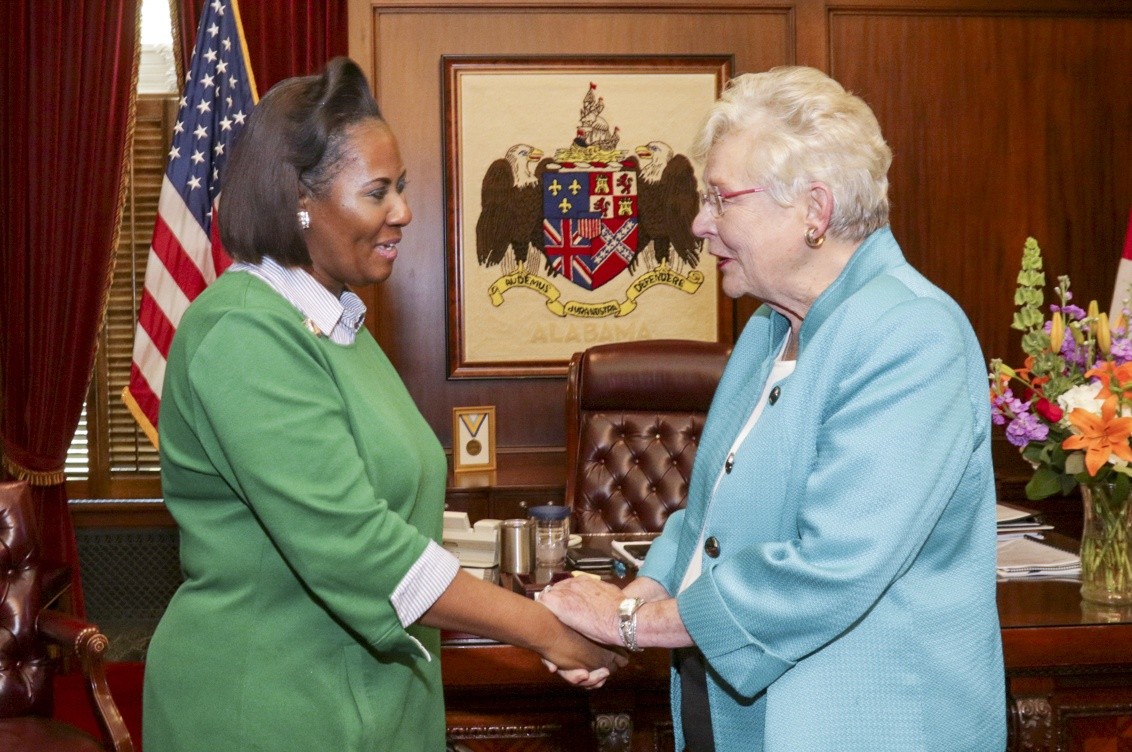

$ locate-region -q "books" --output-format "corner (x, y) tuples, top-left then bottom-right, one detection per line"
(997, 522), (1081, 577)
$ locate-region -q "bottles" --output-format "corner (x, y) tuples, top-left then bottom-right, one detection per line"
(529, 505), (571, 567)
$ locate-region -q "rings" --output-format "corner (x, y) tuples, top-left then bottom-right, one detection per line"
(544, 584), (552, 592)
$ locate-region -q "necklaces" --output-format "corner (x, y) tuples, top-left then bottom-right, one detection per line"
(783, 334), (791, 361)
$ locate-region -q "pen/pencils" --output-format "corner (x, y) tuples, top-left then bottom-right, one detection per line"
(614, 561), (626, 571)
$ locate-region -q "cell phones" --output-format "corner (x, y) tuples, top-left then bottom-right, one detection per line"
(625, 545), (652, 560)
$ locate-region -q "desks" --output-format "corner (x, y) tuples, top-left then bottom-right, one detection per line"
(442, 533), (1132, 752)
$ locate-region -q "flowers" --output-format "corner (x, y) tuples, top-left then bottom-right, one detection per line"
(988, 237), (1132, 593)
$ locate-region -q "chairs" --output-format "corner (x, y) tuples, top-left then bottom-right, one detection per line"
(0, 481), (135, 752)
(564, 340), (734, 532)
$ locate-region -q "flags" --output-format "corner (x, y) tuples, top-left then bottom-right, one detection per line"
(120, 0), (258, 448)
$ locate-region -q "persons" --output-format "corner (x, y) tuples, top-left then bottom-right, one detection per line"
(534, 67), (1007, 752)
(142, 58), (631, 752)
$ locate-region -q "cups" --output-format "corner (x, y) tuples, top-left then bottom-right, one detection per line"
(501, 518), (536, 574)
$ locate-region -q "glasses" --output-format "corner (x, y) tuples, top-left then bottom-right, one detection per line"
(697, 184), (770, 218)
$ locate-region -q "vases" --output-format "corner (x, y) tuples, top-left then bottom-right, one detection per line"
(1078, 482), (1132, 605)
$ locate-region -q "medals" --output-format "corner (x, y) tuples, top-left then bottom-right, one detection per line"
(466, 439), (482, 455)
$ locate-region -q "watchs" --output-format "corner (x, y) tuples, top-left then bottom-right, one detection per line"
(618, 595), (647, 653)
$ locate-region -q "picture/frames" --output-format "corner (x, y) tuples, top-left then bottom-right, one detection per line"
(452, 405), (495, 473)
(439, 52), (738, 381)
